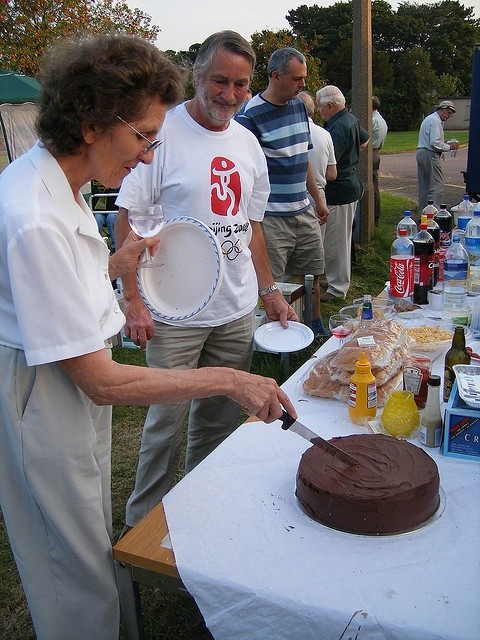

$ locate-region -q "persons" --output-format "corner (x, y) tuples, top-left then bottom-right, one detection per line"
(91, 183), (120, 253)
(299, 90), (337, 242)
(114, 28), (299, 538)
(314, 85), (370, 301)
(0, 34), (297, 640)
(372, 96), (387, 226)
(415, 101), (459, 221)
(231, 47), (329, 368)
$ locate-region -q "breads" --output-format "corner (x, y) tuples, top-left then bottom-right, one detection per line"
(301, 319), (410, 408)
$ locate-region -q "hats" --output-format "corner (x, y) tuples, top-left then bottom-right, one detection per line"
(436, 101), (456, 113)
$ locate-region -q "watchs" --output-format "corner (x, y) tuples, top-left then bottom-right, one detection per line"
(258, 284), (278, 297)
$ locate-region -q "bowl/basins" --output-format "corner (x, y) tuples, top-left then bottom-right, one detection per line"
(353, 296), (395, 311)
(427, 288), (443, 312)
(339, 303), (398, 332)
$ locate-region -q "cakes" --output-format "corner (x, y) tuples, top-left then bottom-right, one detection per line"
(295, 434), (440, 535)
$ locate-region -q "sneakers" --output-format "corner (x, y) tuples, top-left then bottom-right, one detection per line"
(319, 281), (328, 288)
(312, 319), (326, 336)
(320, 292), (333, 302)
(312, 334), (330, 349)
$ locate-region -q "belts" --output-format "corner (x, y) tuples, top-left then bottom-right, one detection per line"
(418, 147), (427, 149)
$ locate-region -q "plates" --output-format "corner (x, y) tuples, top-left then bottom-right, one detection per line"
(465, 340), (480, 361)
(135, 214), (225, 324)
(254, 319), (314, 354)
(402, 324), (475, 351)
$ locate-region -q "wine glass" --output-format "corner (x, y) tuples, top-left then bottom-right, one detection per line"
(329, 314), (354, 347)
(127, 203), (166, 269)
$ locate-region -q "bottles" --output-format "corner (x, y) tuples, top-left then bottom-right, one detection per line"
(381, 389), (420, 439)
(347, 350), (378, 425)
(442, 325), (472, 404)
(457, 194), (477, 248)
(396, 210), (419, 244)
(417, 375), (445, 447)
(463, 209), (480, 308)
(441, 234), (470, 324)
(400, 352), (432, 411)
(410, 223), (437, 305)
(359, 294), (375, 330)
(420, 211), (440, 288)
(389, 228), (415, 310)
(433, 203), (453, 247)
(419, 199), (439, 225)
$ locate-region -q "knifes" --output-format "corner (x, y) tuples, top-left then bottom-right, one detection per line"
(276, 408), (361, 468)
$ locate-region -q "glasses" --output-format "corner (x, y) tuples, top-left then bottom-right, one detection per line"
(115, 114), (163, 153)
